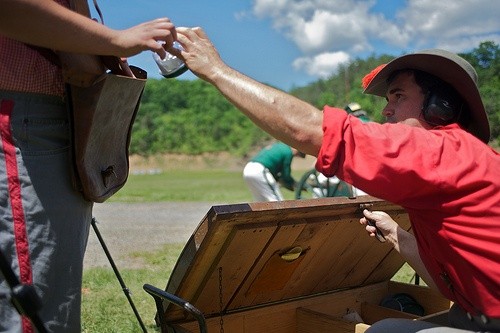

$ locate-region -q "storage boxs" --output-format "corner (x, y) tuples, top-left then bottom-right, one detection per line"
(143, 196), (454, 333)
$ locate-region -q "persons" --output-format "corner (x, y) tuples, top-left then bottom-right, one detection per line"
(0, 0), (177, 333)
(162, 27), (500, 333)
(243, 141), (307, 201)
(344, 102), (374, 196)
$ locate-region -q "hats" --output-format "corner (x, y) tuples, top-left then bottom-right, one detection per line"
(363, 49), (491, 142)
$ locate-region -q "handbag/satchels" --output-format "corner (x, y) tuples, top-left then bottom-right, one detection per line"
(73, 65), (147, 202)
